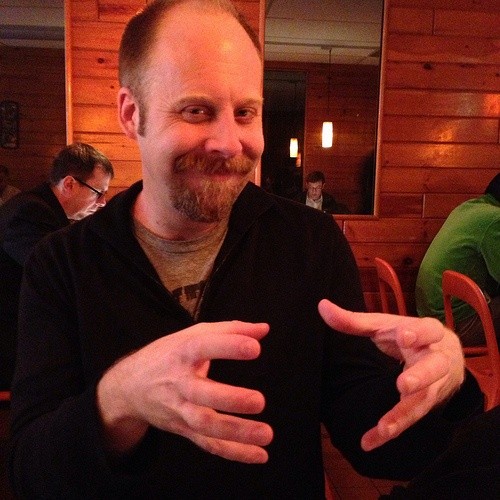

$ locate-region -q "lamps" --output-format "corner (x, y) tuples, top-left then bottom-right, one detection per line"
(290, 82), (299, 159)
(321, 46), (334, 149)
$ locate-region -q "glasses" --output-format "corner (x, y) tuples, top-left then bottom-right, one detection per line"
(61, 176), (107, 200)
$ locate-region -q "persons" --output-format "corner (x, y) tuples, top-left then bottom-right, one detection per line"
(293, 171), (334, 209)
(1, 141), (115, 392)
(13, 1), (486, 498)
(414, 169), (500, 338)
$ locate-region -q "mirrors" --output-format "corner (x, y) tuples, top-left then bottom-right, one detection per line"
(254, 1), (389, 221)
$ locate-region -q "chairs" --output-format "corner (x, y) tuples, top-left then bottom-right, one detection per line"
(441, 270), (500, 414)
(374, 257), (408, 315)
